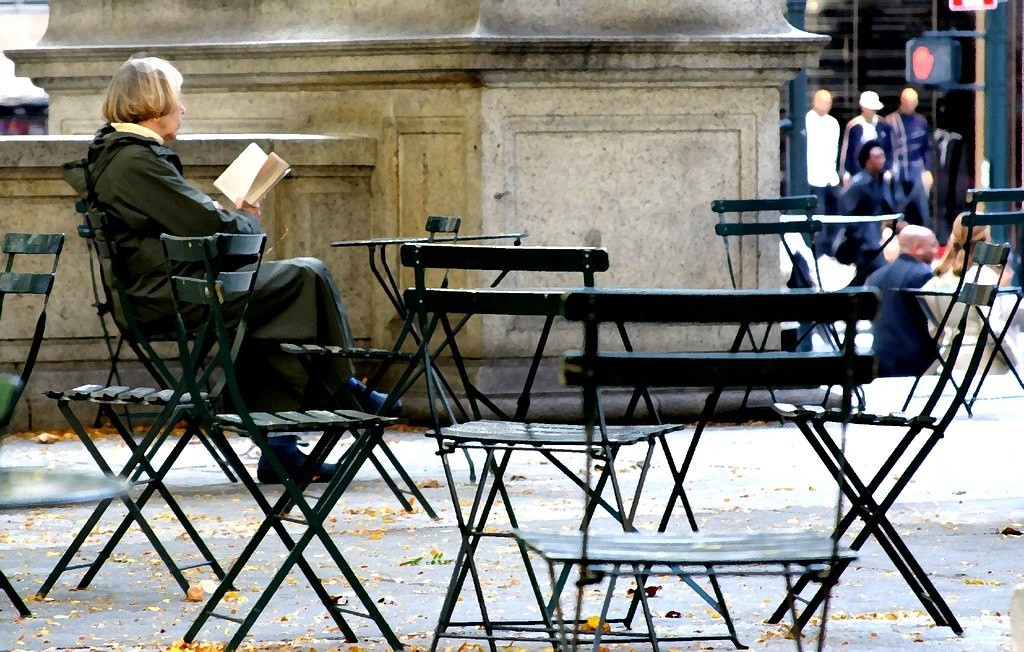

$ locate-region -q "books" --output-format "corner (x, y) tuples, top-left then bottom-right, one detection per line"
(213, 142), (291, 209)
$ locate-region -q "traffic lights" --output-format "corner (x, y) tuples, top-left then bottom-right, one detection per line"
(905, 39), (961, 88)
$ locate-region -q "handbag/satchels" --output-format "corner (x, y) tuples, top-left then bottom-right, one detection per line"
(832, 229), (859, 264)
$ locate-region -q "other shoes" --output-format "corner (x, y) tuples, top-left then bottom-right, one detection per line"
(258, 448), (341, 485)
(306, 374), (402, 415)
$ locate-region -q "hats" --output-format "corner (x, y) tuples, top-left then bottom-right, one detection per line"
(858, 90), (885, 111)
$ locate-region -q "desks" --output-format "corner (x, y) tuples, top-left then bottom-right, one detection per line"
(780, 211), (902, 412)
(330, 229), (523, 482)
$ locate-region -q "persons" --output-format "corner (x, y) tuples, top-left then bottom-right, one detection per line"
(87, 56), (402, 484)
(840, 141), (909, 272)
(806, 90), (840, 253)
(886, 87), (932, 226)
(923, 213), (1015, 375)
(864, 225), (940, 378)
(839, 91), (892, 187)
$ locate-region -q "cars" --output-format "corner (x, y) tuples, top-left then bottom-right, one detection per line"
(782, 213), (1017, 373)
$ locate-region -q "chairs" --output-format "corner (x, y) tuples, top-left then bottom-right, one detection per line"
(1, 176), (1023, 650)
